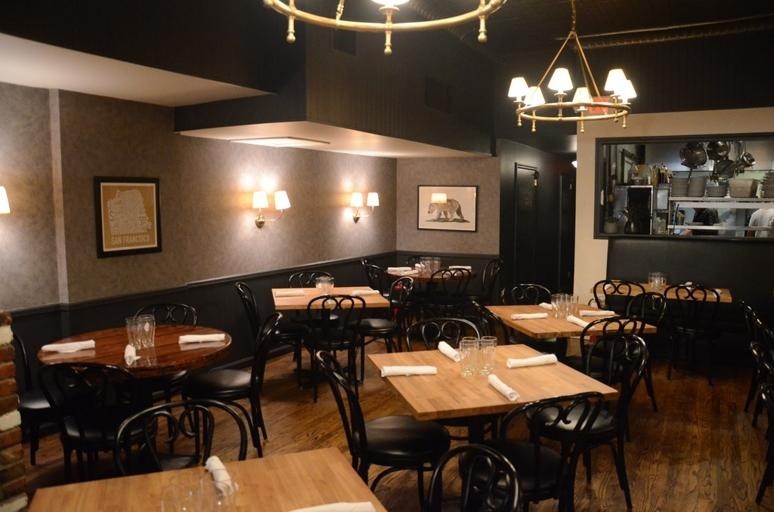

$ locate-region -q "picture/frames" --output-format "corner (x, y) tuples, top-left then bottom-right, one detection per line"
(417, 184), (478, 233)
(622, 148), (638, 185)
(592, 132), (774, 242)
(93, 178), (161, 259)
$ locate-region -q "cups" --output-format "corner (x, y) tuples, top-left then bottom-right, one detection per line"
(551, 293), (579, 319)
(648, 272), (667, 291)
(162, 470), (239, 512)
(316, 276), (334, 297)
(459, 335), (497, 377)
(128, 315), (154, 349)
(421, 257), (441, 275)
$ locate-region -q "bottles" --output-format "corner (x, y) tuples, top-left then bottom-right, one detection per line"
(628, 161), (670, 185)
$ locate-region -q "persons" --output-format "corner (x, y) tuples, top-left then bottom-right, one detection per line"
(679, 208), (719, 236)
(747, 208), (774, 238)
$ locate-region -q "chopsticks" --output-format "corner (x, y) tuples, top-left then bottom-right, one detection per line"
(670, 171), (727, 197)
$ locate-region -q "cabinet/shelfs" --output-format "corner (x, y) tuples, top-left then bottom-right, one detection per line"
(666, 196), (773, 238)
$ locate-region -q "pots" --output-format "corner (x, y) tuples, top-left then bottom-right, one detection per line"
(680, 141), (756, 178)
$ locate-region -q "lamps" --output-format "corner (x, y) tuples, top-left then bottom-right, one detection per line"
(260, 1), (512, 52)
(351, 192), (379, 223)
(507, 1), (639, 132)
(0, 186), (11, 214)
(253, 190), (291, 228)
(638, 163), (652, 186)
